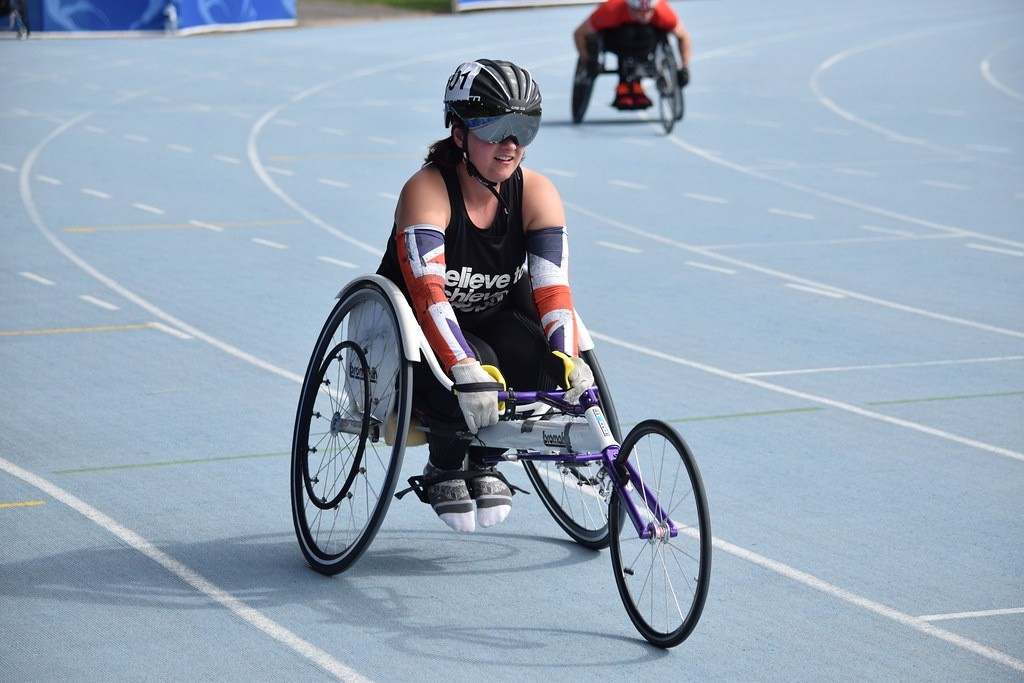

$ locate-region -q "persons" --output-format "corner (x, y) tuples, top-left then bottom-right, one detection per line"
(573, 0), (693, 110)
(375, 58), (594, 531)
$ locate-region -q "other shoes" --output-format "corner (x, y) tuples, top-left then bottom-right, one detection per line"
(616, 83), (633, 107)
(631, 81), (652, 106)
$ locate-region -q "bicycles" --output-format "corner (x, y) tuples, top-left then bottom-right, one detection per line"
(571, 31), (684, 134)
(292, 275), (711, 649)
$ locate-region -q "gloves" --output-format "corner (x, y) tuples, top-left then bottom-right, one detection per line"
(563, 357), (595, 405)
(451, 361), (499, 435)
(585, 58), (599, 79)
(678, 68), (688, 87)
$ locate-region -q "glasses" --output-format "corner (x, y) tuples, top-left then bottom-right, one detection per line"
(458, 100), (543, 147)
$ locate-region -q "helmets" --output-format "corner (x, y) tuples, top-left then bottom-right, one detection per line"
(444, 58), (543, 128)
(626, 0), (658, 10)
